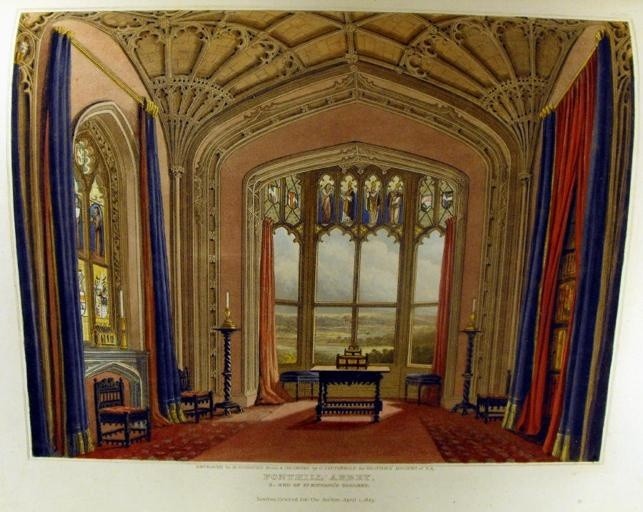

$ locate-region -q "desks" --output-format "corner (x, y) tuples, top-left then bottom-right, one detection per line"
(310, 365), (391, 422)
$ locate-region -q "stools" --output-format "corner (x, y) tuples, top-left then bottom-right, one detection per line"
(404, 373), (443, 407)
(279, 370), (320, 401)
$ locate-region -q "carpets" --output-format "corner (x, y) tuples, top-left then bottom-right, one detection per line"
(416, 413), (563, 463)
(73, 420), (254, 461)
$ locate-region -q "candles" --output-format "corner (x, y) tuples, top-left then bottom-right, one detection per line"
(472, 297), (476, 312)
(225, 289), (230, 310)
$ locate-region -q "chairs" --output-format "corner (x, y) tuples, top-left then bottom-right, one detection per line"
(476, 369), (513, 424)
(93, 376), (152, 448)
(176, 366), (214, 424)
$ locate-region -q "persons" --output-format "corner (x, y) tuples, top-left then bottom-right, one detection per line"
(74, 197), (82, 249)
(89, 206), (104, 258)
(267, 180), (453, 227)
